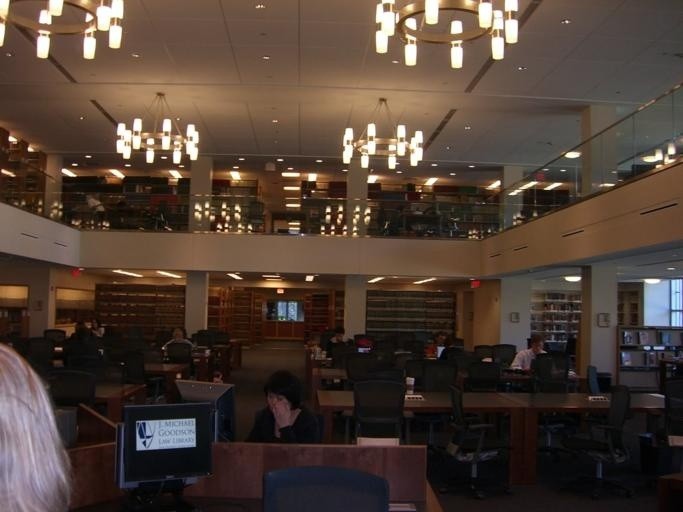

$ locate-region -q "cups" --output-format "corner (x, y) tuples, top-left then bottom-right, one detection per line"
(406, 378), (415, 394)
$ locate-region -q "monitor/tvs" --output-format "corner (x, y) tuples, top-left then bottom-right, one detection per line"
(120, 401), (214, 481)
(171, 378), (237, 442)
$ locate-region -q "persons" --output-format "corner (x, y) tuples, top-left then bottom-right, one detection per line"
(423, 331), (448, 357)
(85, 193), (106, 215)
(441, 337), (463, 359)
(243, 369), (319, 444)
(0, 343), (76, 512)
(327, 327), (353, 351)
(208, 369), (225, 384)
(163, 328), (195, 348)
(509, 334), (548, 371)
(385, 204), (406, 234)
(90, 317), (105, 338)
(566, 326), (577, 355)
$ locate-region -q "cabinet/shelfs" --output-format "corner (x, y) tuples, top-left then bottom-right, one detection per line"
(532, 290), (638, 343)
(303, 289), (457, 342)
(96, 284), (263, 346)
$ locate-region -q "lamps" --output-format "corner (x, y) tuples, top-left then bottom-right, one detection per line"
(115, 93), (199, 165)
(0, 1), (124, 60)
(374, 0), (518, 69)
(342, 98), (423, 169)
